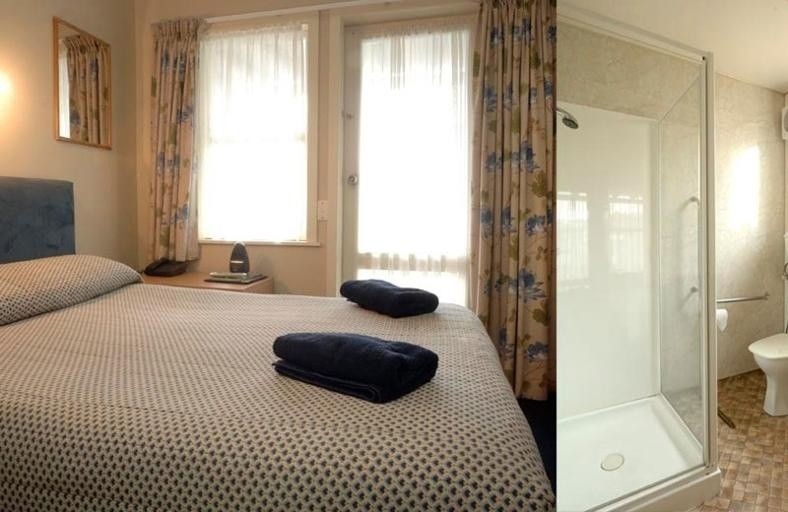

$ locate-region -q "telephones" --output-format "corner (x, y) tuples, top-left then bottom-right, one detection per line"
(144, 258), (187, 276)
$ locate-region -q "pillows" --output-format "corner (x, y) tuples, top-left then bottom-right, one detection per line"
(0, 253), (141, 325)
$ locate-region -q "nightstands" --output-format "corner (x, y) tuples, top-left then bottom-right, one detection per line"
(135, 271), (273, 294)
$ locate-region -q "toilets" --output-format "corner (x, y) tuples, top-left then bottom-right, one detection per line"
(747, 333), (788, 417)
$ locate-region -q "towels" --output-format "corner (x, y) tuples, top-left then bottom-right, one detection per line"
(270, 332), (441, 404)
(341, 278), (441, 318)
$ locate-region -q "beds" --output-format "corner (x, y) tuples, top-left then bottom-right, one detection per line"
(0, 176), (557, 512)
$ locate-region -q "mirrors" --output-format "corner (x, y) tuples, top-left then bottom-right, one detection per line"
(53, 16), (112, 152)
